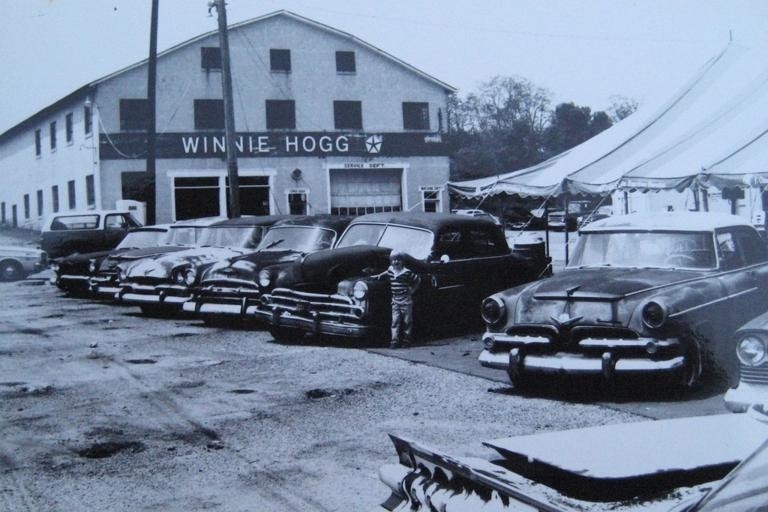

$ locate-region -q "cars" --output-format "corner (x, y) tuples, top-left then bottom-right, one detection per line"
(90, 222), (180, 310)
(479, 213), (767, 393)
(183, 213), (360, 327)
(117, 213), (290, 320)
(255, 207), (555, 341)
(50, 251), (117, 294)
(451, 198), (615, 235)
(378, 412), (766, 512)
(721, 310), (768, 412)
(0, 244), (49, 282)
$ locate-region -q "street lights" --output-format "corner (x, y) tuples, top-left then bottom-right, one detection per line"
(205, 0), (241, 219)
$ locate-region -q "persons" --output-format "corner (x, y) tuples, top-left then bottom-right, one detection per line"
(370, 249), (422, 349)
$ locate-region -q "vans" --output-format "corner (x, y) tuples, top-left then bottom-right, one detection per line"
(42, 206), (145, 258)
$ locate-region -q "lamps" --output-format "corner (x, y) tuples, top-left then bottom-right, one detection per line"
(83, 94), (93, 108)
